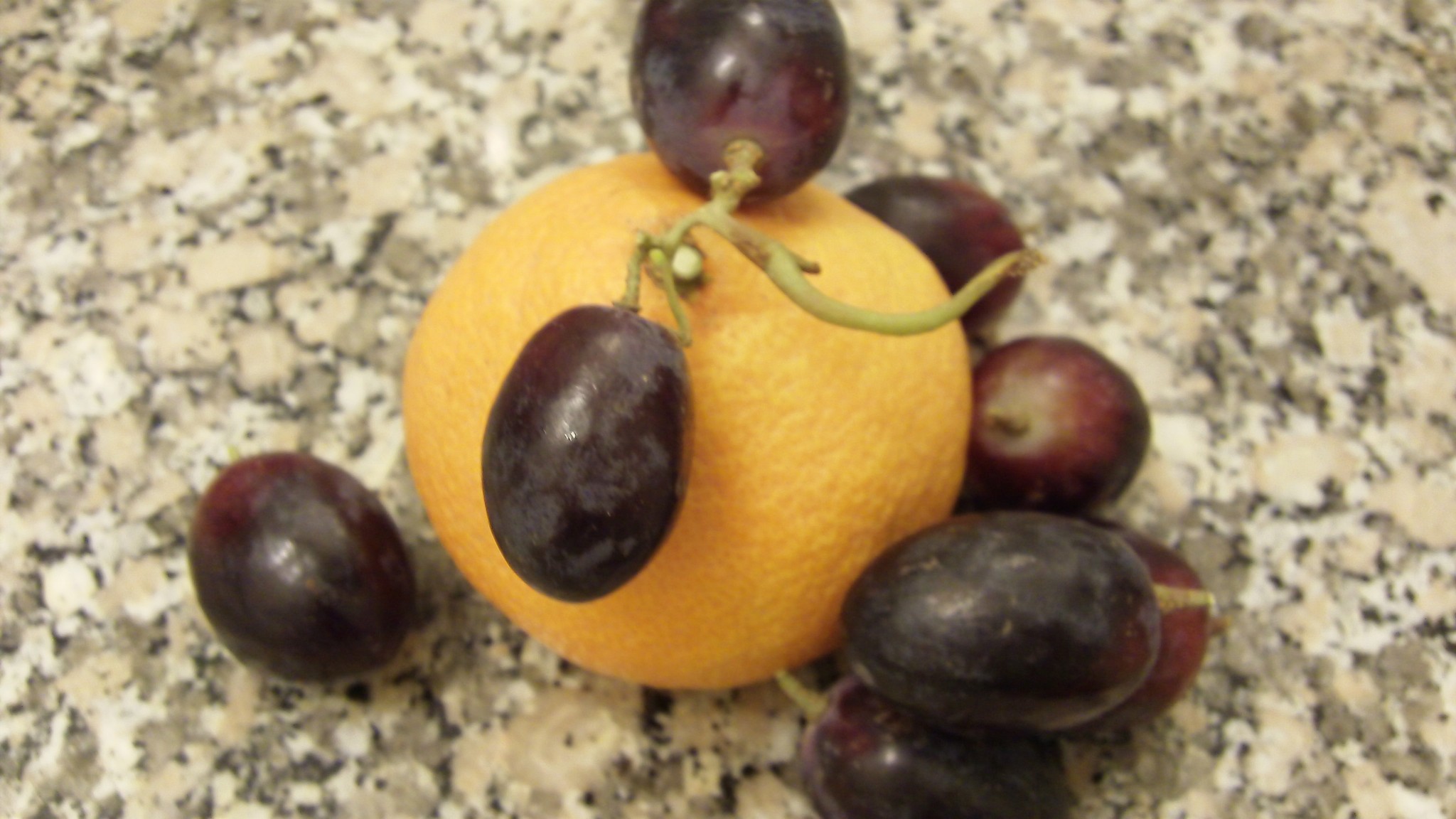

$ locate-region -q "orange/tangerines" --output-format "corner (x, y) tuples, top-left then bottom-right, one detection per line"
(404, 153), (971, 689)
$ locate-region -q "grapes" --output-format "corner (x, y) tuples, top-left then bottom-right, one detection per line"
(480, 4), (1211, 819)
(188, 448), (413, 680)
